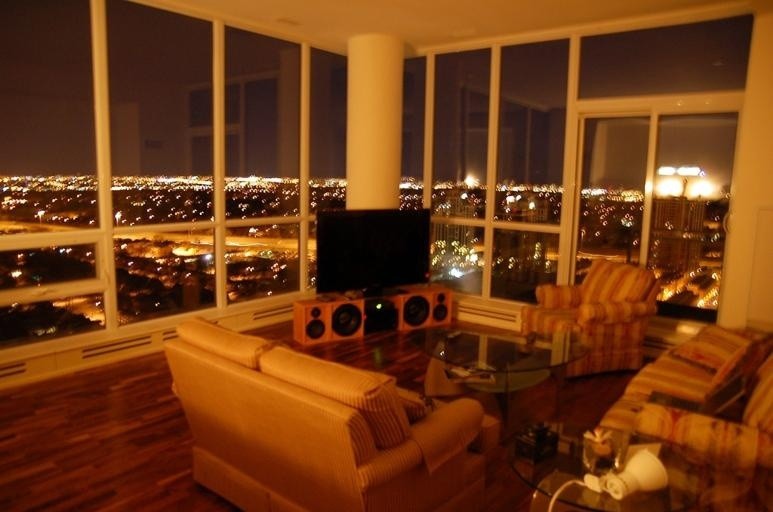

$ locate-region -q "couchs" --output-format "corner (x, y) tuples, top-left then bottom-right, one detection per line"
(517, 258), (661, 378)
(601, 324), (773, 512)
(163, 318), (501, 512)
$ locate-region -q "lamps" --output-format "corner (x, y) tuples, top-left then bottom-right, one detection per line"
(608, 450), (669, 500)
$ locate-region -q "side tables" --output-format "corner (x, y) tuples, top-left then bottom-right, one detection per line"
(503, 449), (682, 512)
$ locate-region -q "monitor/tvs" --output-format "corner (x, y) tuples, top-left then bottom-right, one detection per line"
(316, 209), (430, 297)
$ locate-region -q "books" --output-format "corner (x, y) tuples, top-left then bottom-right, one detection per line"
(451, 360), (496, 378)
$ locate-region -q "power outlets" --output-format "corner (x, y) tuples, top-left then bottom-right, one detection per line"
(584, 471), (616, 493)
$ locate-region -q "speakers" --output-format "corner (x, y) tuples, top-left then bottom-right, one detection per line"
(293, 298), (365, 346)
(393, 285), (453, 332)
